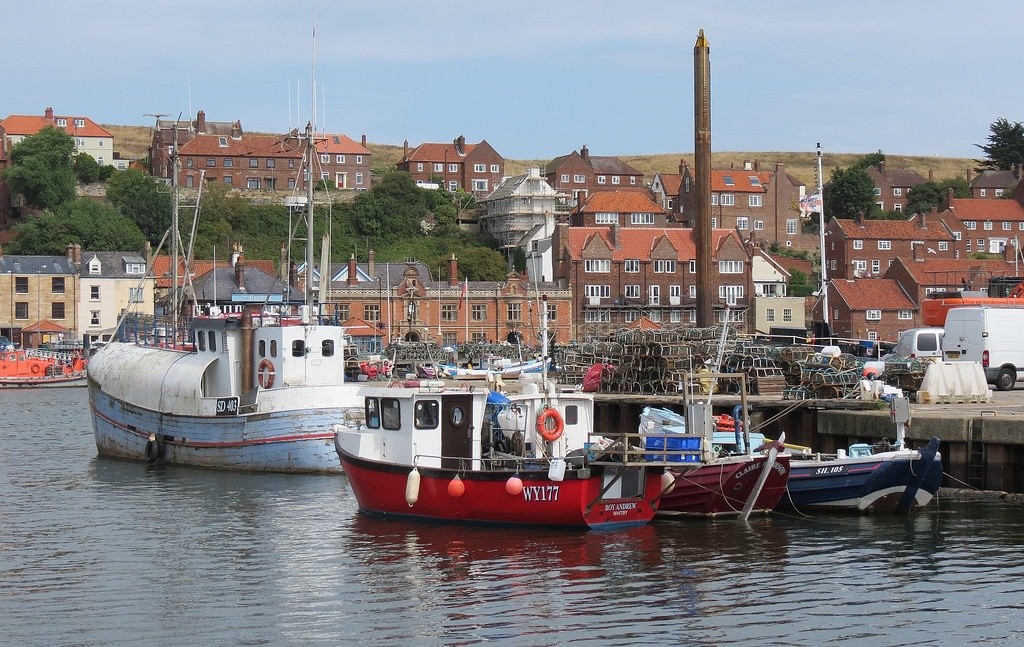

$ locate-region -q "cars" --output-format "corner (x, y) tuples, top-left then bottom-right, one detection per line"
(55, 339), (96, 352)
(0, 336), (11, 350)
(89, 341), (108, 350)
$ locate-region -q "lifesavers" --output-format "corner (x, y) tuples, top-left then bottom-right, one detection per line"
(144, 439), (160, 463)
(257, 358), (276, 390)
(712, 414), (744, 433)
(535, 407), (565, 442)
(30, 363), (40, 373)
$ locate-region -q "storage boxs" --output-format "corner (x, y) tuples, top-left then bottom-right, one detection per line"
(645, 437), (702, 462)
(584, 442), (595, 459)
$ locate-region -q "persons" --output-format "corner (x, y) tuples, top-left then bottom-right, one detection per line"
(468, 357), (473, 370)
(381, 360), (390, 378)
(451, 356), (455, 363)
(203, 303), (211, 316)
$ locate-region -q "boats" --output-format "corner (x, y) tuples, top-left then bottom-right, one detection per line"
(84, 111), (367, 475)
(332, 370), (665, 530)
(774, 437), (945, 515)
(656, 430), (791, 518)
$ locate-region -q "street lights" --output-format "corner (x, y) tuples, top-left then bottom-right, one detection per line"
(813, 143), (828, 325)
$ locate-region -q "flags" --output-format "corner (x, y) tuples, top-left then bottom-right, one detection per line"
(456, 282), (466, 311)
(800, 195), (821, 212)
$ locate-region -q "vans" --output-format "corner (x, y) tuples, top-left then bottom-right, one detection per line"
(894, 328), (945, 366)
(941, 306), (1024, 390)
